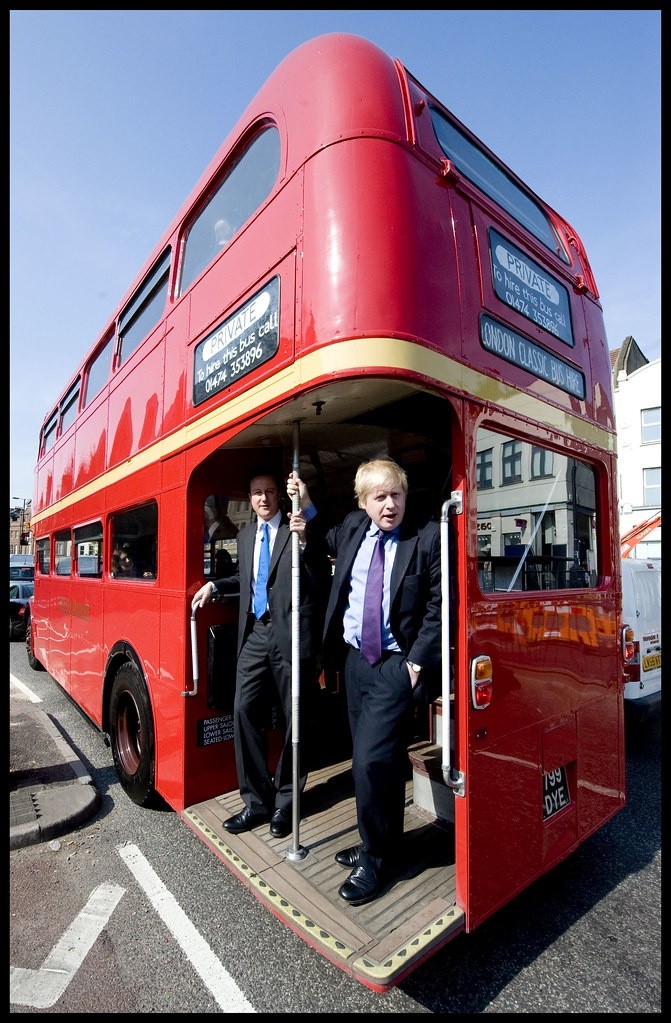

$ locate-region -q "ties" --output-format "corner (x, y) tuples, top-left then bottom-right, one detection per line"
(254, 522), (270, 621)
(360, 529), (392, 667)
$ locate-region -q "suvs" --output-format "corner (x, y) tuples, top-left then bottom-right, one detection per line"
(620, 559), (662, 717)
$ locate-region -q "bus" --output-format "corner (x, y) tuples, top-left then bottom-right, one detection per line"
(29, 31), (628, 993)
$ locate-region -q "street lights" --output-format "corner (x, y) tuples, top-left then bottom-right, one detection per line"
(13, 496), (25, 554)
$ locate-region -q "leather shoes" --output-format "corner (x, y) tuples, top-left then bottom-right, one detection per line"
(269, 808), (293, 838)
(222, 805), (274, 832)
(338, 856), (395, 906)
(334, 841), (365, 870)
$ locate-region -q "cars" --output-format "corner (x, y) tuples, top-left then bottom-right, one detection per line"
(57, 556), (101, 574)
(10, 552), (33, 640)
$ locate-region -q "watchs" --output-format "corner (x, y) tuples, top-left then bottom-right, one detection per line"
(407, 660), (421, 672)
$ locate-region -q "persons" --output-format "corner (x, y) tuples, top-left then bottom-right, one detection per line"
(191, 468), (334, 837)
(286, 456), (452, 905)
(203, 494), (242, 541)
(113, 542), (234, 579)
